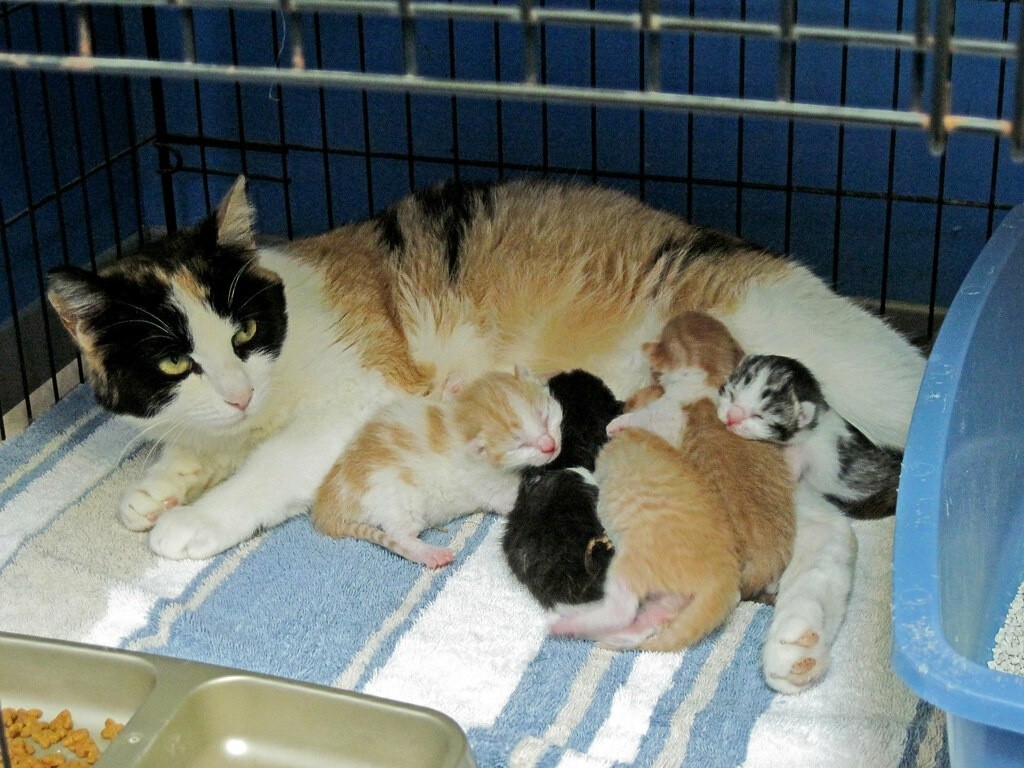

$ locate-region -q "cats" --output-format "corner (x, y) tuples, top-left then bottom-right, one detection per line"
(44, 173), (928, 695)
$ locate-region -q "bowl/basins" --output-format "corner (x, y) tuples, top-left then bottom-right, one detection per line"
(0, 630), (476, 768)
